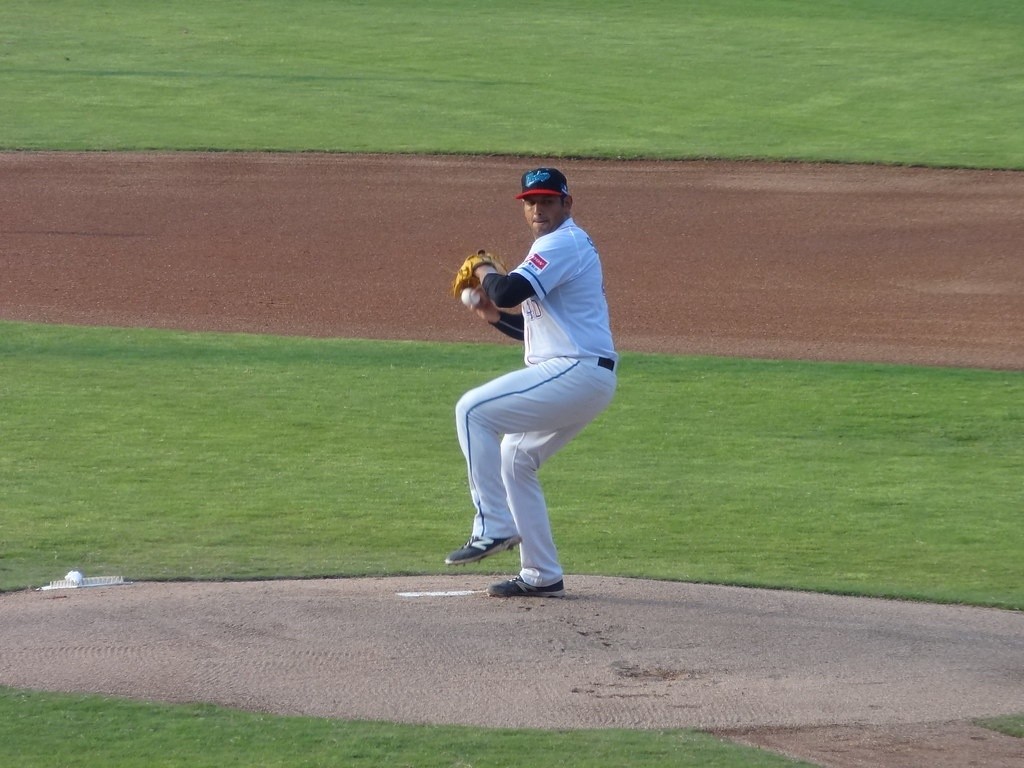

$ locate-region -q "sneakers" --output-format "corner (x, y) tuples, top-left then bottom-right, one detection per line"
(445, 534), (522, 565)
(489, 574), (565, 597)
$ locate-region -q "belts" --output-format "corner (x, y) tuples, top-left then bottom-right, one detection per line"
(598, 357), (615, 371)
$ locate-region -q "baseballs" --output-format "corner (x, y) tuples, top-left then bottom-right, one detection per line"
(461, 287), (480, 307)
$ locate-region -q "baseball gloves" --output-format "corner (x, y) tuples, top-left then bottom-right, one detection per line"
(453, 250), (508, 301)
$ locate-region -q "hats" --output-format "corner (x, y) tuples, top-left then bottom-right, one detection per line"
(517, 168), (568, 199)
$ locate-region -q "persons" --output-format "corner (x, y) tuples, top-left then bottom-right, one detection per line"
(445, 168), (619, 598)
